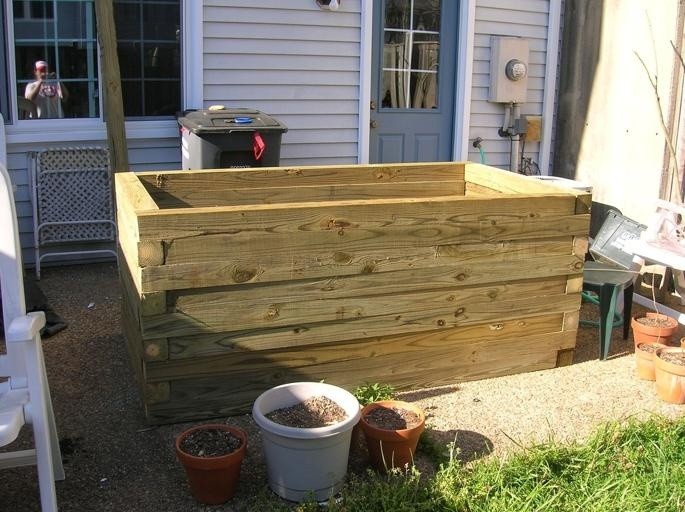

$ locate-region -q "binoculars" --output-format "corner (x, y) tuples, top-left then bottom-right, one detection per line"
(40, 72), (56, 79)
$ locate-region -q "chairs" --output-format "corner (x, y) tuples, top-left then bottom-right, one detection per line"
(1, 169), (66, 512)
(30, 146), (112, 282)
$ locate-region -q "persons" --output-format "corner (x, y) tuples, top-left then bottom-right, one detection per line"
(24, 60), (70, 119)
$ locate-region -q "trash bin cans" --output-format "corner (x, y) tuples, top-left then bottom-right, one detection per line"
(176, 108), (288, 168)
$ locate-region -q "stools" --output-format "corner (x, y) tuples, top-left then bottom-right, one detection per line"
(582, 262), (633, 362)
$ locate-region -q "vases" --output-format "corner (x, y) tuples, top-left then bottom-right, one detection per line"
(175, 424), (247, 505)
(361, 400), (425, 472)
(629, 312), (685, 405)
(252, 381), (361, 502)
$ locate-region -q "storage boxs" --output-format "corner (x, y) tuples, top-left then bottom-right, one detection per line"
(108, 161), (592, 427)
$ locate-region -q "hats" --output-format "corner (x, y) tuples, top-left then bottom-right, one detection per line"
(34, 61), (49, 71)
